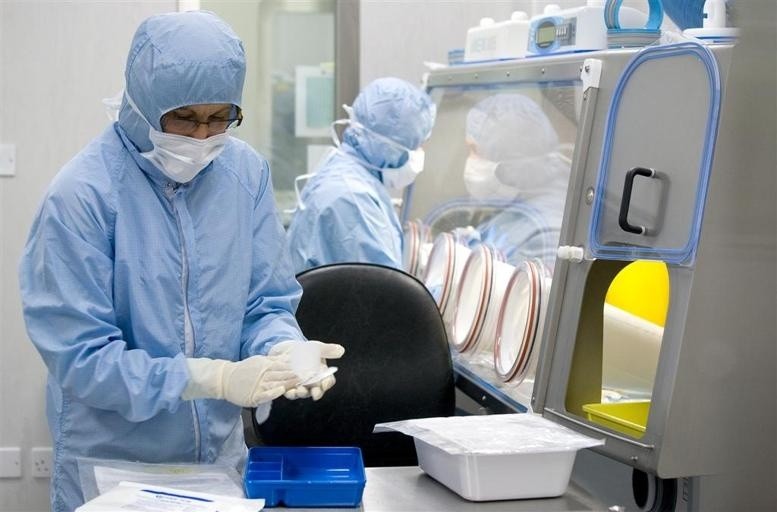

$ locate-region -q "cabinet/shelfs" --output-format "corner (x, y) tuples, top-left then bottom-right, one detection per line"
(398, 3), (776, 512)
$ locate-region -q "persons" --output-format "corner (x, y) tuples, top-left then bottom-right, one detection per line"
(285, 76), (437, 275)
(18, 8), (345, 512)
(450, 93), (572, 277)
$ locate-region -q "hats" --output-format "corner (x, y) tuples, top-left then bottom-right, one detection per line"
(118, 11), (245, 185)
(464, 94), (564, 195)
(343, 78), (437, 181)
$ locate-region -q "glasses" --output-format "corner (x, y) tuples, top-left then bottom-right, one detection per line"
(159, 108), (244, 135)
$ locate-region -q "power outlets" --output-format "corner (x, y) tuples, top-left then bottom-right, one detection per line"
(31, 447), (54, 478)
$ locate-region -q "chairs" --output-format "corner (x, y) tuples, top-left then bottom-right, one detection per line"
(244, 264), (455, 469)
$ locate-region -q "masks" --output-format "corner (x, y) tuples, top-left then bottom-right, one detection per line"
(463, 155), (559, 205)
(346, 119), (425, 190)
(116, 93), (232, 183)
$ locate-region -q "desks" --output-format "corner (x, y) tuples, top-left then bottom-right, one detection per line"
(254, 466), (618, 512)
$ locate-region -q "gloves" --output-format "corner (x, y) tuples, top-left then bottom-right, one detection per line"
(179, 356), (303, 408)
(268, 339), (345, 400)
